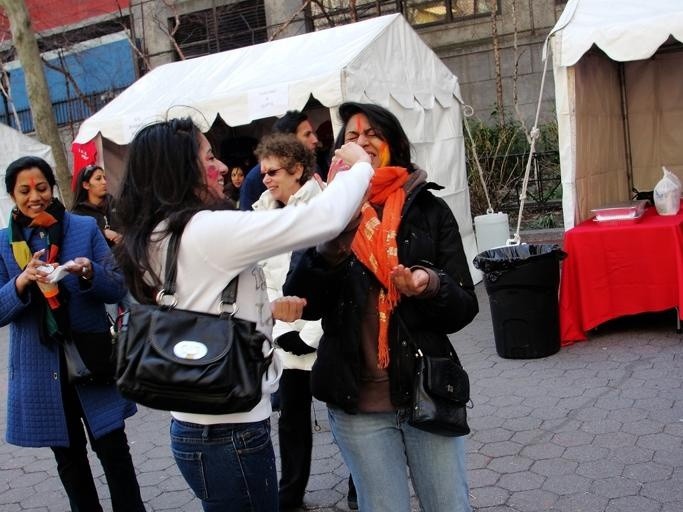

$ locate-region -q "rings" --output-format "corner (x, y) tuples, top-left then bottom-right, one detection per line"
(82, 267), (88, 273)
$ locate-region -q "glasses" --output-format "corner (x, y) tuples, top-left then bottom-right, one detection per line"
(258, 165), (283, 181)
(82, 164), (101, 177)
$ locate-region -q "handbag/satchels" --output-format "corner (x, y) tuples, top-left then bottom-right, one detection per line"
(406, 349), (474, 439)
(112, 294), (275, 414)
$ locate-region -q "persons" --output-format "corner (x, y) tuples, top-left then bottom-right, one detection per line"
(0, 154), (147, 511)
(235, 110), (319, 208)
(223, 163), (249, 196)
(115, 118), (372, 512)
(68, 165), (140, 321)
(250, 130), (358, 512)
(280, 101), (479, 511)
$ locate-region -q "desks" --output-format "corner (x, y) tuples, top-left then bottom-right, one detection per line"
(557, 205), (682, 346)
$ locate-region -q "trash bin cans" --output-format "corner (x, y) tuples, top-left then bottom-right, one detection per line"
(477, 243), (562, 360)
